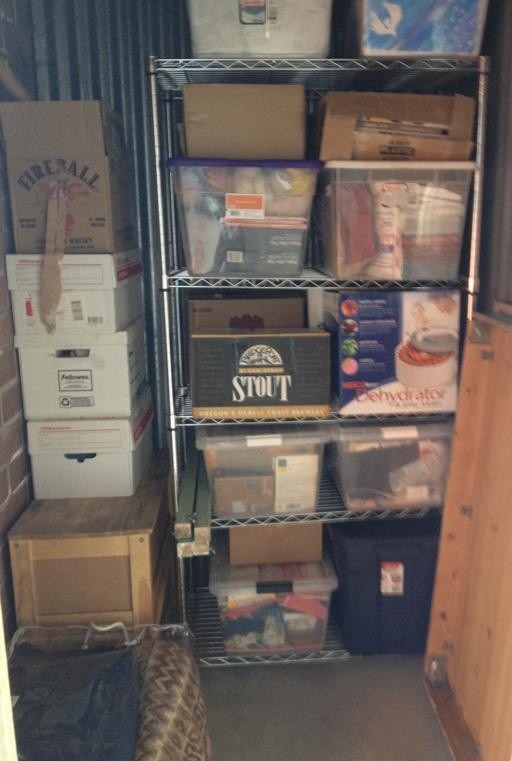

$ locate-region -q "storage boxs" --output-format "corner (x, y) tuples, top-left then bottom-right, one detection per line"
(8, 494), (163, 632)
(5, 252), (157, 500)
(1, 101), (139, 255)
(195, 425), (331, 518)
(190, 289), (461, 419)
(209, 522), (436, 656)
(328, 157), (476, 280)
(165, 157), (323, 277)
(331, 421), (454, 511)
(178, 84), (307, 159)
(319, 90), (476, 163)
